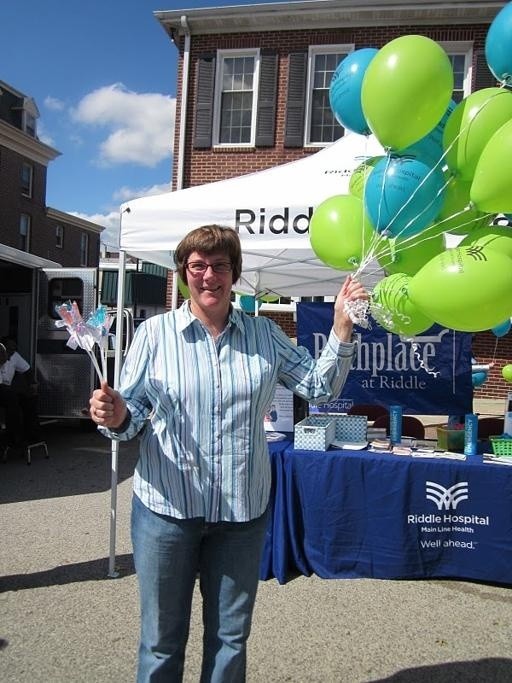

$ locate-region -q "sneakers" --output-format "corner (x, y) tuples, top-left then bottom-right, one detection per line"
(0, 444), (12, 466)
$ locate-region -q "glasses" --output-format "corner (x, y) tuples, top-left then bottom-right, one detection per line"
(182, 260), (235, 274)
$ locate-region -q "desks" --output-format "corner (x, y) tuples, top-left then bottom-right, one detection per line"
(281, 434), (512, 594)
(255, 431), (296, 587)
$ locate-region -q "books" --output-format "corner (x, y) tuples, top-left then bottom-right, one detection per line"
(367, 427), (438, 457)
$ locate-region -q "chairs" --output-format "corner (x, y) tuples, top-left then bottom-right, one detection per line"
(346, 402), (390, 427)
(370, 413), (426, 441)
(475, 418), (505, 442)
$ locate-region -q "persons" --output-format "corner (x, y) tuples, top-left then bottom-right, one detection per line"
(1, 332), (33, 451)
(471, 333), (494, 390)
(84, 224), (375, 683)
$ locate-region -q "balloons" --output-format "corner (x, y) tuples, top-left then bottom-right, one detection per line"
(501, 363), (512, 380)
(308, 1), (512, 338)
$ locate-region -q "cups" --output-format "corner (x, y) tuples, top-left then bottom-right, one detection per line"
(463, 414), (478, 456)
(389, 406), (402, 444)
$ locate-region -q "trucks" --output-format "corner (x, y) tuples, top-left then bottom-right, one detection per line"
(0, 242), (135, 465)
